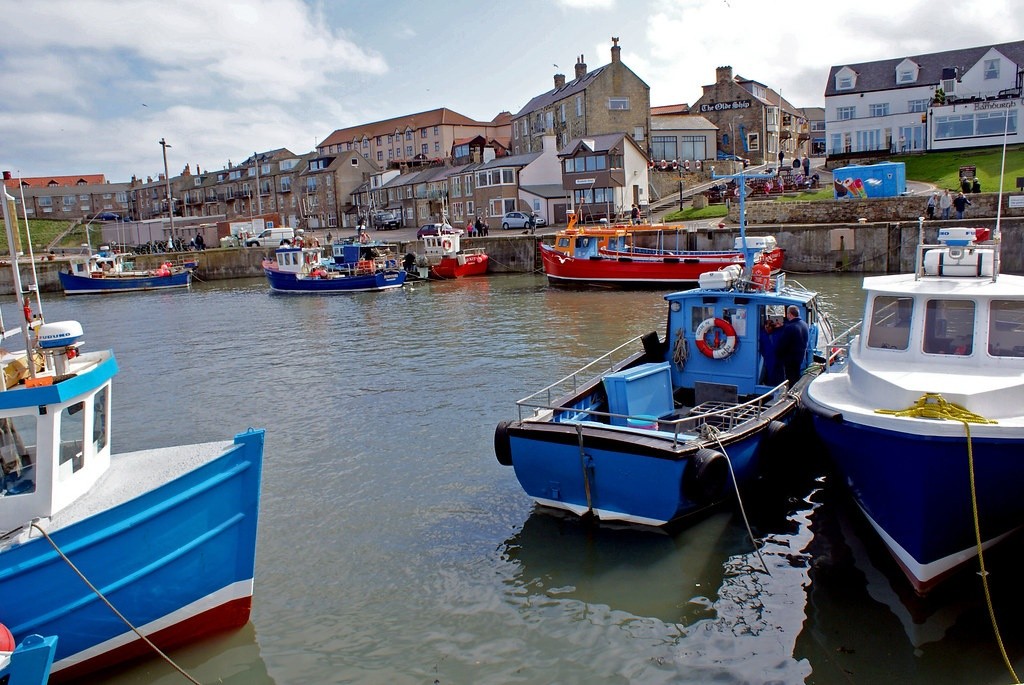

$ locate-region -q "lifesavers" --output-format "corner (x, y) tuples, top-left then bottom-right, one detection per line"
(365, 233), (371, 241)
(566, 229), (579, 234)
(443, 240), (451, 250)
(829, 347), (842, 364)
(359, 234), (366, 242)
(306, 253), (316, 264)
(695, 318), (737, 360)
(309, 237), (319, 247)
(294, 235), (303, 247)
(682, 448), (729, 506)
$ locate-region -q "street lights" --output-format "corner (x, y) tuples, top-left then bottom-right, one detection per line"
(158, 137), (175, 243)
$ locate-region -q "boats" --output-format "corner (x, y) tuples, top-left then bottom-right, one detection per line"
(0, 171), (266, 685)
(537, 162), (786, 291)
(494, 107), (1024, 685)
(58, 224), (199, 296)
(262, 193), (489, 295)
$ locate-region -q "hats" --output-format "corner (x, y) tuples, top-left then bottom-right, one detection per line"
(961, 177), (967, 180)
(974, 178), (978, 182)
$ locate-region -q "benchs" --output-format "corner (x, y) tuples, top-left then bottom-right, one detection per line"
(701, 174), (819, 203)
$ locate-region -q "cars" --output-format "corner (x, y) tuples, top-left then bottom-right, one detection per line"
(502, 211), (546, 230)
(416, 222), (465, 240)
(100, 212), (120, 221)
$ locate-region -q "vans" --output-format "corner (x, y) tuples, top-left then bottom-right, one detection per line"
(243, 228), (294, 247)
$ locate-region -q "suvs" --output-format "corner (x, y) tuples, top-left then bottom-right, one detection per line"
(372, 212), (400, 231)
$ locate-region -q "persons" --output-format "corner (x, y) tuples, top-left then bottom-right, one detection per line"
(900, 136), (906, 152)
(794, 172), (802, 190)
(405, 252), (416, 272)
(191, 233), (203, 250)
(467, 218), (489, 237)
(954, 192), (971, 219)
(940, 188), (952, 220)
(793, 155), (801, 175)
(760, 305), (808, 389)
(529, 212), (536, 234)
(778, 176), (784, 191)
(779, 151), (784, 167)
(802, 155), (810, 176)
(927, 192), (938, 219)
(712, 182), (727, 197)
(358, 218), (364, 234)
(765, 180), (773, 197)
(631, 204), (640, 225)
(962, 176), (981, 193)
(807, 172), (819, 189)
